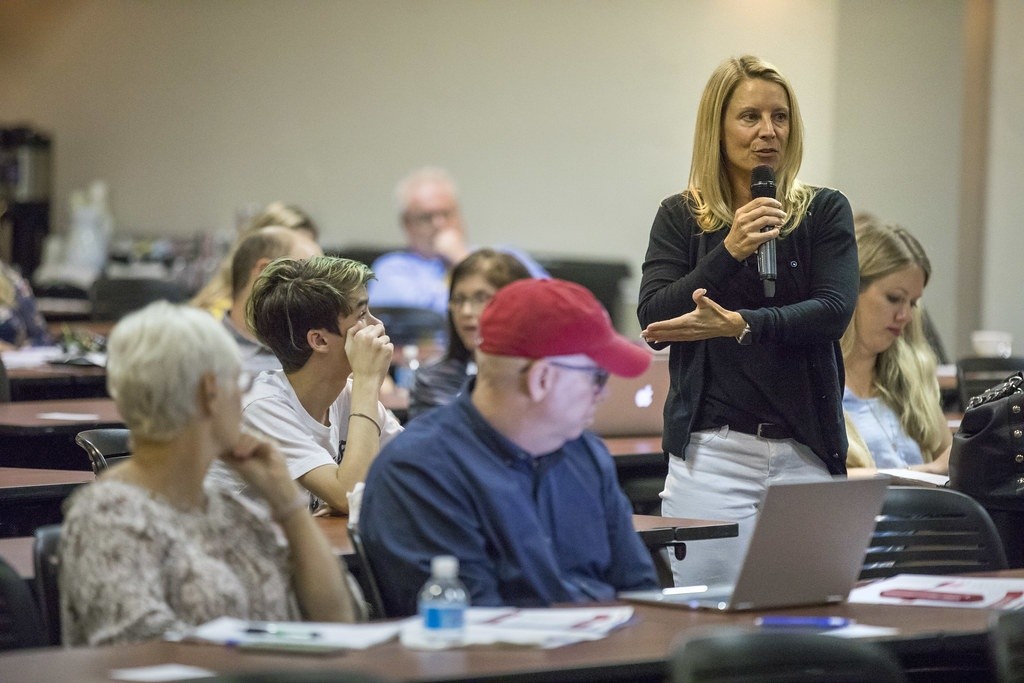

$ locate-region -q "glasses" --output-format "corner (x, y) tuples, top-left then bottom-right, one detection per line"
(518, 355), (610, 391)
(447, 288), (496, 314)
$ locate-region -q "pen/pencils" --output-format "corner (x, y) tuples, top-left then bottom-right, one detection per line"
(754, 615), (854, 628)
(244, 628), (321, 638)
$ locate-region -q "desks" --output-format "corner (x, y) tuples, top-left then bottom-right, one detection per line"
(0, 316), (1024, 683)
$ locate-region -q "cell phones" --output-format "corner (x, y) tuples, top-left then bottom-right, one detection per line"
(753, 617), (847, 628)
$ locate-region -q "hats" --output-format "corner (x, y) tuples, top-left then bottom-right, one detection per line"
(478, 277), (651, 378)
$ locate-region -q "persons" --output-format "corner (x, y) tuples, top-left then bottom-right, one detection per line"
(367, 171), (553, 383)
(223, 228), (324, 356)
(407, 251), (531, 420)
(839, 214), (952, 476)
(638, 53), (860, 587)
(360, 278), (652, 604)
(61, 302), (361, 643)
(205, 258), (406, 520)
(0, 260), (52, 352)
(192, 202), (318, 316)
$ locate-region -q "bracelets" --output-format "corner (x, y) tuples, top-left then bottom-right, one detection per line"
(349, 414), (381, 436)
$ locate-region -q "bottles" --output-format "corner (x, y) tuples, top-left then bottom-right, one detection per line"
(394, 345), (423, 391)
(417, 556), (470, 649)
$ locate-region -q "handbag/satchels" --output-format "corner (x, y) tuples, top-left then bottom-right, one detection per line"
(948, 369), (1023, 505)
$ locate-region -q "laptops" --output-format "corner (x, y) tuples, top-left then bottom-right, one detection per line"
(586, 360), (670, 435)
(618, 476), (891, 611)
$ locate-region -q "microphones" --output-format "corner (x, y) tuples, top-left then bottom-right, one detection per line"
(750, 165), (776, 297)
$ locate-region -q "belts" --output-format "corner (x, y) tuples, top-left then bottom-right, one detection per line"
(727, 419), (796, 440)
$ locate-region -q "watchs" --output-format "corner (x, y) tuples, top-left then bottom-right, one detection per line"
(736, 323), (752, 344)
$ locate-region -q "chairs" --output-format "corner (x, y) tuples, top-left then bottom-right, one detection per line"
(853, 488), (1023, 683)
